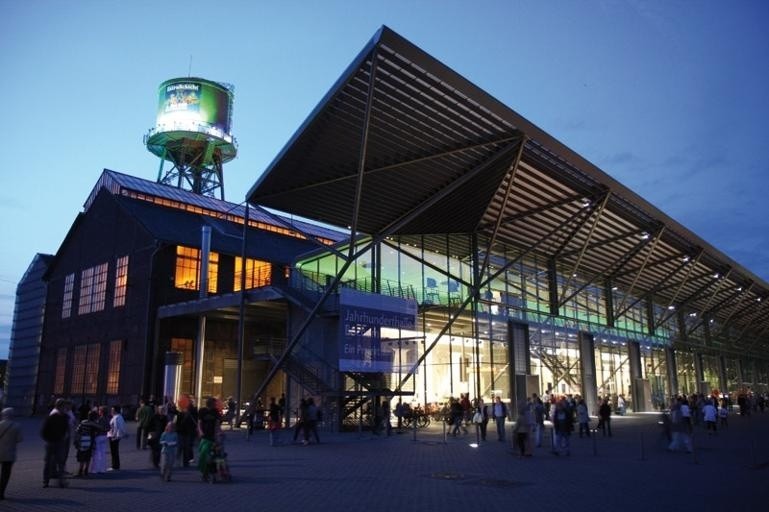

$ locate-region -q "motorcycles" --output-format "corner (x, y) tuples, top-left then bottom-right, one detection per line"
(433, 402), (450, 423)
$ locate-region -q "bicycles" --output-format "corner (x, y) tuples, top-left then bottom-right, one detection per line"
(401, 404), (430, 428)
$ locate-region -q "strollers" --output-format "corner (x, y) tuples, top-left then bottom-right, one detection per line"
(200, 434), (232, 485)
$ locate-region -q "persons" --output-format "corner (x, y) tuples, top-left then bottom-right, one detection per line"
(34, 395), (323, 489)
(444, 391), (626, 458)
(1, 407), (22, 498)
(667, 390), (768, 454)
